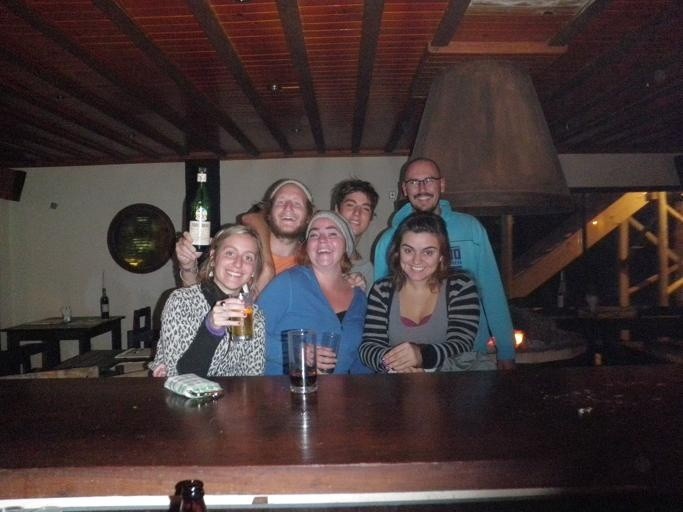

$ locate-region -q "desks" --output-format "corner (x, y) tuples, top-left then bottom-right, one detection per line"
(0, 316), (125, 375)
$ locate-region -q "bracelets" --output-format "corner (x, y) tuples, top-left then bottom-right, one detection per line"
(179, 259), (198, 283)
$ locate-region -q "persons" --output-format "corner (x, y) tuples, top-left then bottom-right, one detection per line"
(374, 158), (516, 372)
(358, 211), (480, 374)
(252, 210), (376, 375)
(330, 177), (380, 297)
(147, 224), (266, 377)
(177, 179), (367, 291)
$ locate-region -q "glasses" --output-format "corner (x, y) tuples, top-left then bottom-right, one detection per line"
(405, 177), (442, 185)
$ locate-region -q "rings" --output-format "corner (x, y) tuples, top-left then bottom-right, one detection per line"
(221, 301), (225, 306)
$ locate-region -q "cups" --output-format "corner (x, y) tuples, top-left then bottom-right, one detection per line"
(321, 331), (340, 376)
(288, 330), (318, 395)
(63, 304), (71, 324)
(230, 292), (254, 341)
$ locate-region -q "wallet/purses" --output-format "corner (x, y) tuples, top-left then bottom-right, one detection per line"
(164, 373), (224, 399)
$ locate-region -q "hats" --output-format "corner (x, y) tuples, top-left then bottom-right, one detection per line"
(306, 210), (356, 258)
(264, 178), (313, 202)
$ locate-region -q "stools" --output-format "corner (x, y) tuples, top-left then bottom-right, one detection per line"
(0, 344), (46, 375)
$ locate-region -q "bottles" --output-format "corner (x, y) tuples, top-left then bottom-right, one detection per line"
(188, 166), (214, 254)
(173, 480), (207, 511)
(100, 288), (109, 319)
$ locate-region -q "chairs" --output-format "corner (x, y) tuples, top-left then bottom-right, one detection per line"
(127, 307), (151, 348)
